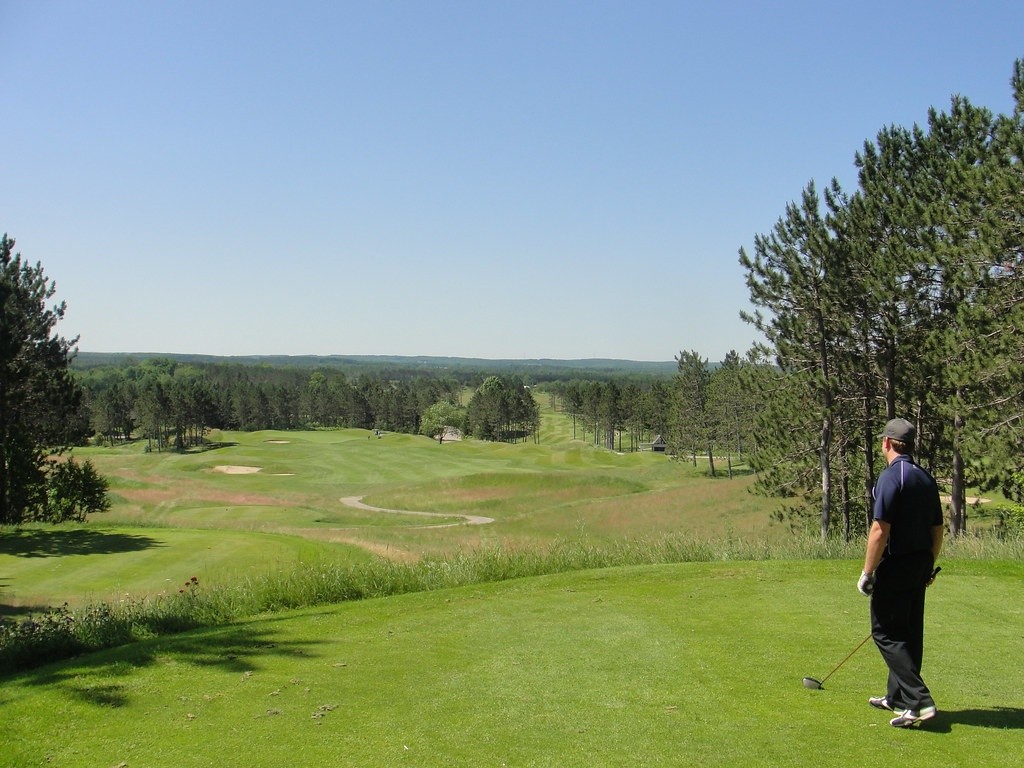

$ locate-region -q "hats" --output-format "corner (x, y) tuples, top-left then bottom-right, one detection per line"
(877, 418), (917, 444)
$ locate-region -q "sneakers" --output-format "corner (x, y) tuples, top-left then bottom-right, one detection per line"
(890, 704), (936, 726)
(869, 696), (905, 716)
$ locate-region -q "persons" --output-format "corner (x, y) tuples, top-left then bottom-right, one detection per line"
(857, 418), (944, 726)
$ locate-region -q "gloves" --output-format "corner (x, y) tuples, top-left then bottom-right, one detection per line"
(926, 569), (935, 587)
(857, 571), (874, 596)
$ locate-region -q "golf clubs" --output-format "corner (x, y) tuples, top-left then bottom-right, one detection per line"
(801, 566), (944, 688)
